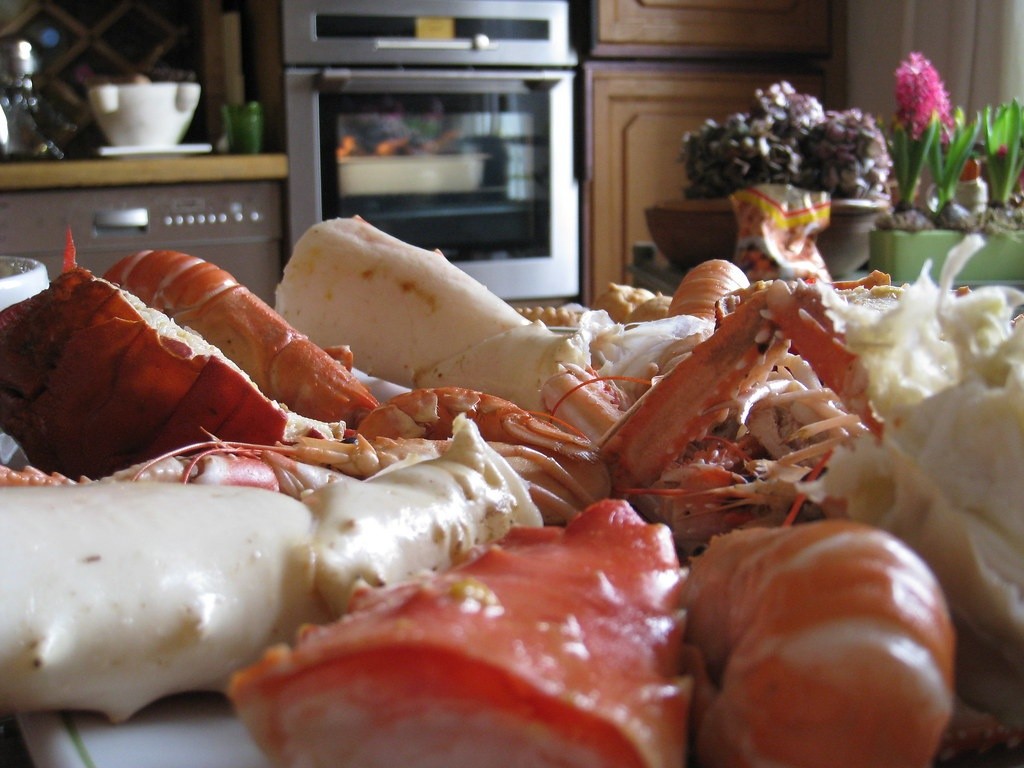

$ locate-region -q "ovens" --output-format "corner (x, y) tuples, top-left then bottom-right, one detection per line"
(268, 1), (585, 302)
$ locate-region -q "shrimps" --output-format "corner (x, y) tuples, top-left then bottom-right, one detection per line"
(667, 258), (751, 326)
(354, 387), (599, 464)
(681, 520), (955, 768)
(100, 250), (380, 432)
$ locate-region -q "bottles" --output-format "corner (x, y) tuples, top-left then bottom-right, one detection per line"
(221, 101), (264, 155)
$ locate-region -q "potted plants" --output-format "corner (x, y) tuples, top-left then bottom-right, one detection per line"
(644, 79), (895, 283)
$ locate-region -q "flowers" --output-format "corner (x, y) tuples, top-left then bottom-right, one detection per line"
(867, 50), (1024, 230)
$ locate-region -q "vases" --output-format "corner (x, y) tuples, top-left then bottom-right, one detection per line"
(869, 228), (1024, 283)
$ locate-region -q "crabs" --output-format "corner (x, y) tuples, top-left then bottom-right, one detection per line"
(1, 214), (1022, 768)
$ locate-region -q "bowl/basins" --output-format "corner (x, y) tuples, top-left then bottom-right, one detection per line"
(88, 81), (202, 145)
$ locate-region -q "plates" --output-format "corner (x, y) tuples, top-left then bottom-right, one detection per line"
(95, 143), (213, 159)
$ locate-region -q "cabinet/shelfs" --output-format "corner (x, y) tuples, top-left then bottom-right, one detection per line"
(0, 151), (287, 309)
(577, 1), (848, 308)
